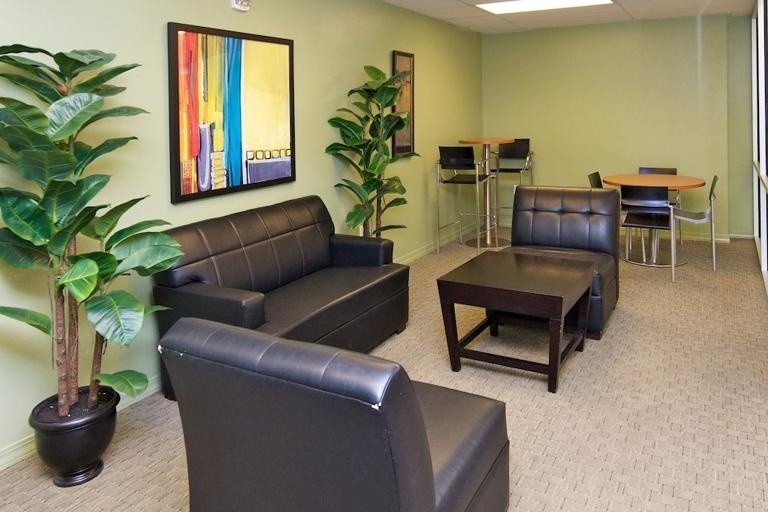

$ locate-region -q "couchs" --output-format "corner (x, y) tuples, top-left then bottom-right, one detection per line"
(153, 195), (409, 403)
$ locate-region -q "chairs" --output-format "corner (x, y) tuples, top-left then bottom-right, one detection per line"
(158, 317), (510, 512)
(489, 139), (534, 243)
(588, 167), (717, 283)
(511, 186), (620, 341)
(433, 146), (498, 255)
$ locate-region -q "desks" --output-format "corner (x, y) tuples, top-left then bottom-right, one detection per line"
(461, 139), (517, 248)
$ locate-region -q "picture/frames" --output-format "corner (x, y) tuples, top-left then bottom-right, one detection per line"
(392, 49), (415, 159)
(167, 22), (296, 202)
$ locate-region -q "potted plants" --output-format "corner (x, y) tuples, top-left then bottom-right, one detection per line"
(0, 43), (183, 489)
